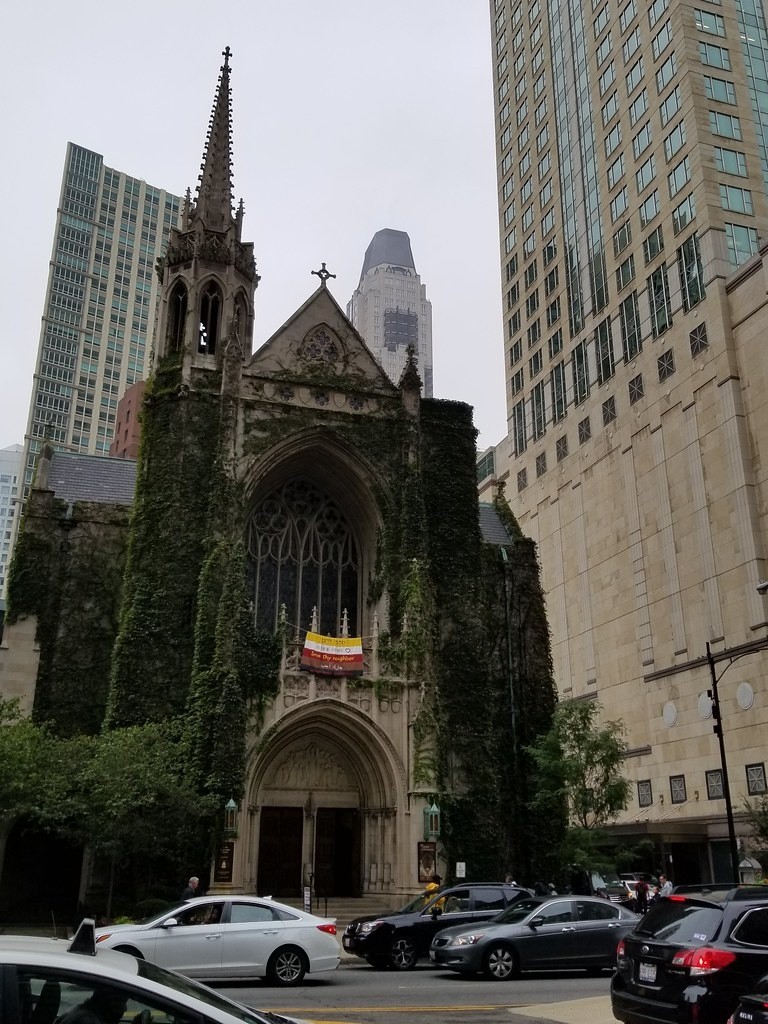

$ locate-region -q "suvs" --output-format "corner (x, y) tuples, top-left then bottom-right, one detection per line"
(610, 883), (768, 1024)
(341, 881), (537, 970)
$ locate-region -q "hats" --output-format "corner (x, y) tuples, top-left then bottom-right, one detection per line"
(432, 875), (443, 880)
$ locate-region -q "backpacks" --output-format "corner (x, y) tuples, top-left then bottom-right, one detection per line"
(637, 882), (645, 898)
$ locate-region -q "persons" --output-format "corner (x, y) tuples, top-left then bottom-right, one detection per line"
(54, 988), (129, 1024)
(622, 881), (629, 896)
(424, 874), (446, 914)
(594, 887), (607, 898)
(548, 883), (558, 895)
(634, 876), (648, 914)
(181, 877), (199, 900)
(650, 875), (673, 904)
(503, 873), (517, 888)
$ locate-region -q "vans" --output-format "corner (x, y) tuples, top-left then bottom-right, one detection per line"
(620, 873), (659, 885)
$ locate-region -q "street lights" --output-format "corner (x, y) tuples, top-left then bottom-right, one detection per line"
(705, 641), (768, 888)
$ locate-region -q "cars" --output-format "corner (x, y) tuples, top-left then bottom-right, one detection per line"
(0, 919), (311, 1024)
(68, 895), (343, 986)
(428, 894), (643, 979)
(613, 881), (655, 903)
